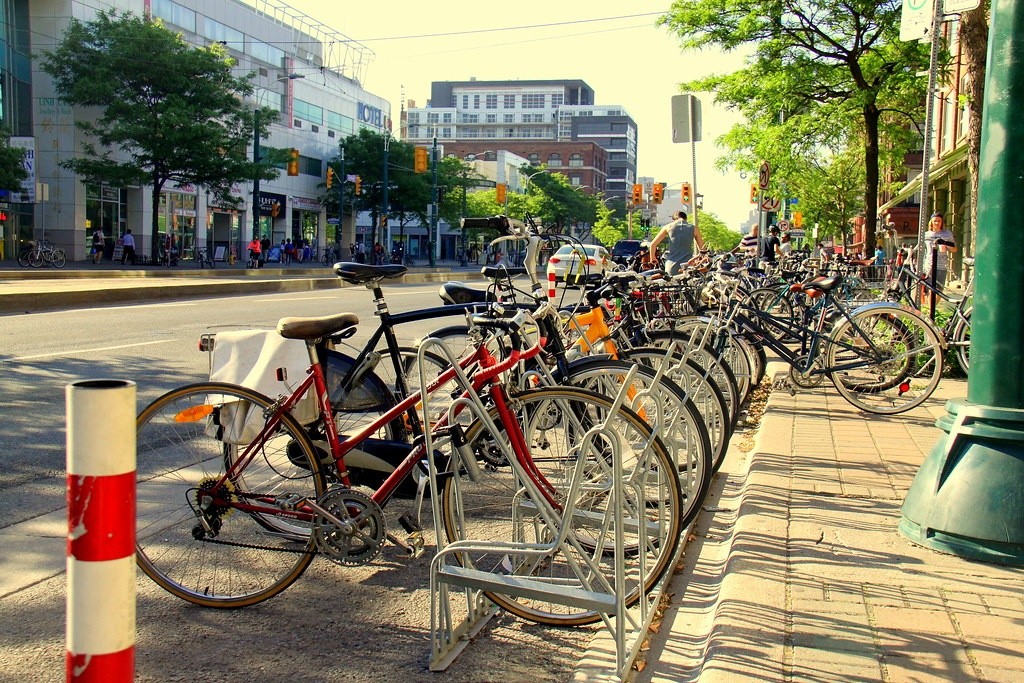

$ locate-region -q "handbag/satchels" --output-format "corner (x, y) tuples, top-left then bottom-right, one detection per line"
(205, 330), (317, 445)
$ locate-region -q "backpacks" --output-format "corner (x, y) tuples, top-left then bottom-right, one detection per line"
(93, 231), (100, 242)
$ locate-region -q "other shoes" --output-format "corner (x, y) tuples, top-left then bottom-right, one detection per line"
(92, 259), (95, 264)
(98, 262), (103, 264)
(121, 259), (125, 265)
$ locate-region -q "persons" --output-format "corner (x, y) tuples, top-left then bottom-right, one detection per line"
(818, 243), (829, 275)
(280, 235), (317, 267)
(738, 224), (759, 266)
(248, 235), (270, 269)
(924, 214), (957, 309)
(895, 243), (908, 284)
(776, 232), (790, 256)
(837, 252), (862, 269)
(649, 212), (707, 277)
(875, 246), (887, 275)
(801, 243), (811, 260)
(458, 247), (516, 265)
(92, 226), (105, 264)
(759, 225), (784, 276)
(349, 241), (399, 265)
(121, 229), (137, 265)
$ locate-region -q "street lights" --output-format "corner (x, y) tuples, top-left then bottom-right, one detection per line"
(524, 171), (549, 227)
(253, 72), (306, 237)
(460, 150), (495, 266)
(384, 123), (421, 261)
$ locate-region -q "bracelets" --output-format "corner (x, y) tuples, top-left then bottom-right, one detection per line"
(700, 251), (707, 254)
(746, 248), (749, 252)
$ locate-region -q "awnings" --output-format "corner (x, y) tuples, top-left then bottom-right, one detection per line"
(877, 133), (970, 214)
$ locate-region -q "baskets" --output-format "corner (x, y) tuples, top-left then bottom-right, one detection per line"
(641, 285), (700, 318)
(839, 264), (888, 309)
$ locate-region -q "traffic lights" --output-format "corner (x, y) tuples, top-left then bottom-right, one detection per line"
(356, 177), (363, 195)
(0, 209), (10, 224)
(750, 184), (759, 204)
(652, 184), (662, 204)
(682, 183), (691, 203)
(633, 184), (642, 203)
(326, 168), (334, 189)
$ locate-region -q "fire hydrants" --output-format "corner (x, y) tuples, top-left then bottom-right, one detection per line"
(228, 255), (235, 265)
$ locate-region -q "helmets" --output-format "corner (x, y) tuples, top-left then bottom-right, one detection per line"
(769, 225), (780, 233)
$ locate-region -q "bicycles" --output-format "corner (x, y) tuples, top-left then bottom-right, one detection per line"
(246, 249), (263, 268)
(18, 239), (66, 269)
(192, 246), (216, 269)
(321, 248), (337, 266)
(135, 222), (975, 626)
